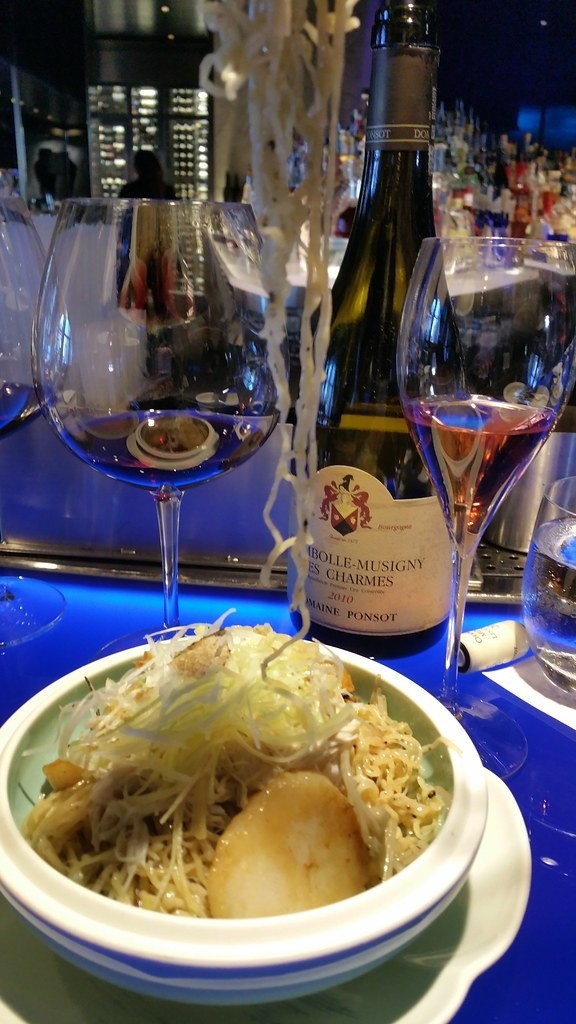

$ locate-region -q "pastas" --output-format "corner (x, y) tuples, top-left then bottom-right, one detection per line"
(191, 0), (361, 689)
(27, 693), (447, 919)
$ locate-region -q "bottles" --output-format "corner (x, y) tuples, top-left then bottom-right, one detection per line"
(287, 0), (467, 660)
(238, 92), (576, 270)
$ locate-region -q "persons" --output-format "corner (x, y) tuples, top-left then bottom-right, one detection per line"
(117, 150), (186, 321)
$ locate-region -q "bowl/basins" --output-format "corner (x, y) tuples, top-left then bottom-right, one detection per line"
(2, 636), (486, 1007)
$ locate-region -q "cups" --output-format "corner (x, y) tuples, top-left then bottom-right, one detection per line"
(517, 474), (576, 703)
(486, 427), (576, 555)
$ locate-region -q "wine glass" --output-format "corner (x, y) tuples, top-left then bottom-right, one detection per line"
(28, 188), (290, 653)
(0, 169), (70, 655)
(399, 233), (576, 780)
